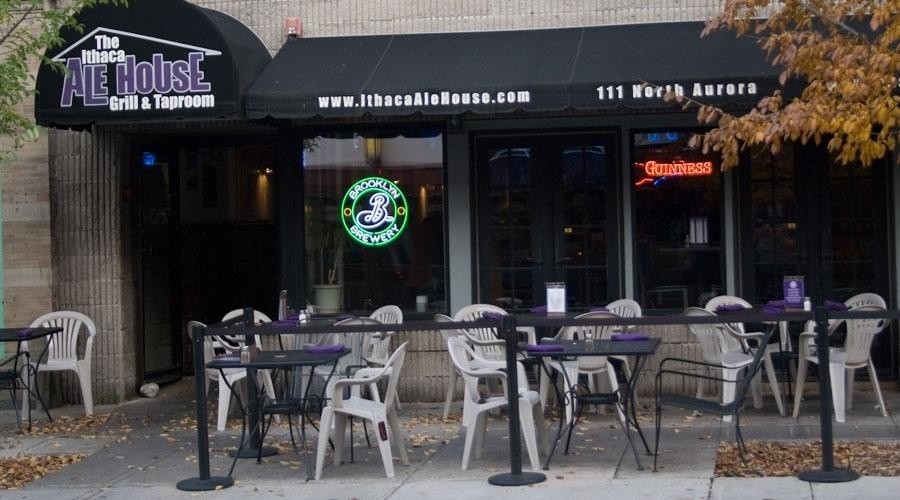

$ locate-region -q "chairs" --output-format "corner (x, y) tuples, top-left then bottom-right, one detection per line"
(18, 311), (97, 419)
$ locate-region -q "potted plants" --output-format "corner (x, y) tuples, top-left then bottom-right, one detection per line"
(306, 198), (353, 314)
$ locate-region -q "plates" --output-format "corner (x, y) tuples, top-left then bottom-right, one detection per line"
(711, 309), (744, 315)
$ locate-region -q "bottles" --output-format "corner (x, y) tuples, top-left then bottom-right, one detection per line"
(243, 345), (251, 362)
(240, 346), (248, 364)
(803, 297), (812, 312)
(278, 289), (288, 322)
(584, 334), (594, 353)
(305, 308), (312, 323)
(298, 310), (307, 325)
(685, 235), (691, 249)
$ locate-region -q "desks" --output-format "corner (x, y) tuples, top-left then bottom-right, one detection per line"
(0, 323), (62, 431)
